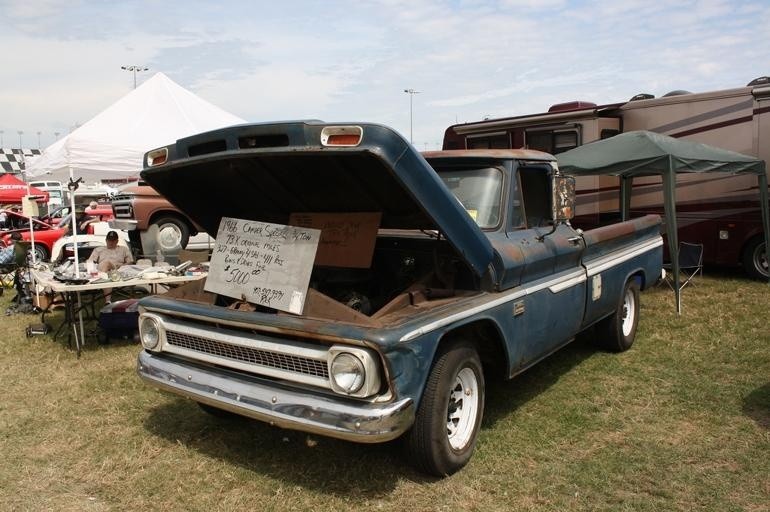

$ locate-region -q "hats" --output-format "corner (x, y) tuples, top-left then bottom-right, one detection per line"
(105, 231), (119, 241)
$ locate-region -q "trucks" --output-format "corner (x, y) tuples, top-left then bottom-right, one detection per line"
(442, 76), (770, 283)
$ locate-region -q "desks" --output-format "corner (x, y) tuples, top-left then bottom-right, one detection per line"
(32, 269), (204, 360)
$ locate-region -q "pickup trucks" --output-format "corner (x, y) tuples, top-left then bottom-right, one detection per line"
(0, 179), (205, 269)
(109, 117), (666, 478)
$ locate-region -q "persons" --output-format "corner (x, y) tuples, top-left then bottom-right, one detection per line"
(85, 201), (101, 221)
(68, 208), (96, 260)
(0, 205), (22, 230)
(90, 230), (134, 306)
(10, 231), (27, 302)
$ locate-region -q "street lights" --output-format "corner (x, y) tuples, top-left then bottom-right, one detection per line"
(121, 64), (149, 90)
(404, 88), (421, 145)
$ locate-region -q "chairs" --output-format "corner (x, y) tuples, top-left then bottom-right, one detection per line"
(655, 241), (707, 294)
